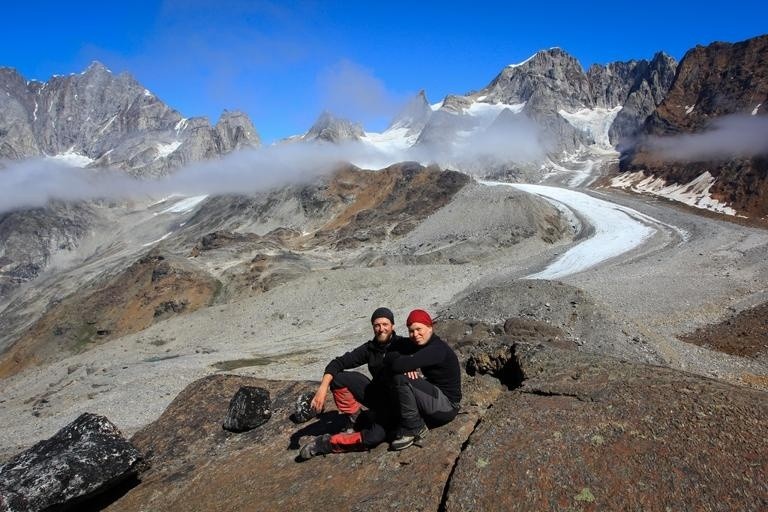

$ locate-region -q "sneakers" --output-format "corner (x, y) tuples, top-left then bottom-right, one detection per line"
(340, 407), (369, 434)
(299, 433), (332, 460)
(391, 422), (430, 451)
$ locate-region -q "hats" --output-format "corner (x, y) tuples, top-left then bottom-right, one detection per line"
(371, 307), (394, 325)
(407, 310), (432, 329)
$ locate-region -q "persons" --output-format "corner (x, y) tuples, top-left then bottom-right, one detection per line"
(300, 307), (413, 460)
(382, 309), (462, 451)
